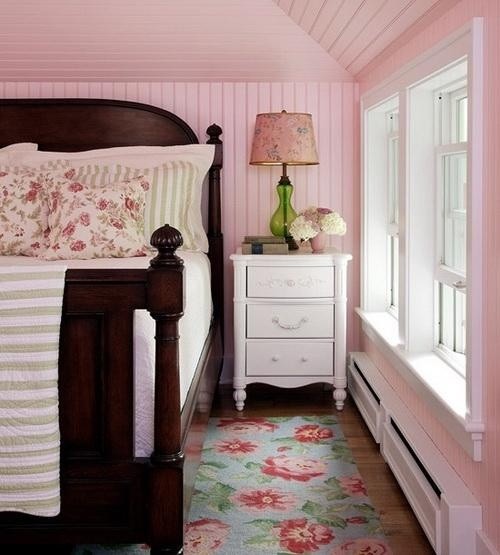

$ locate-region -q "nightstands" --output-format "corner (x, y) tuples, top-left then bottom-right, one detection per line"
(229, 245), (352, 410)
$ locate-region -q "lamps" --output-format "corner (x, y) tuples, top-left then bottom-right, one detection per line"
(250, 106), (322, 238)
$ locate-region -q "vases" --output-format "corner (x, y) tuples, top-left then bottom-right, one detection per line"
(309, 235), (326, 252)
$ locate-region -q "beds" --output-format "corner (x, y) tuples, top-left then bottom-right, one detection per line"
(0, 97), (224, 554)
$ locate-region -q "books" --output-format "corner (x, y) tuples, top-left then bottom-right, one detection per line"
(241, 243), (288, 255)
(244, 236), (286, 243)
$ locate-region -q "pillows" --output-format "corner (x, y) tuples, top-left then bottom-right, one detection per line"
(0, 138), (219, 260)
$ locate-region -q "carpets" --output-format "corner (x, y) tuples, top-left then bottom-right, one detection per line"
(184, 408), (386, 555)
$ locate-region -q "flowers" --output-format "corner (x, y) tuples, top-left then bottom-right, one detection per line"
(282, 202), (349, 245)
